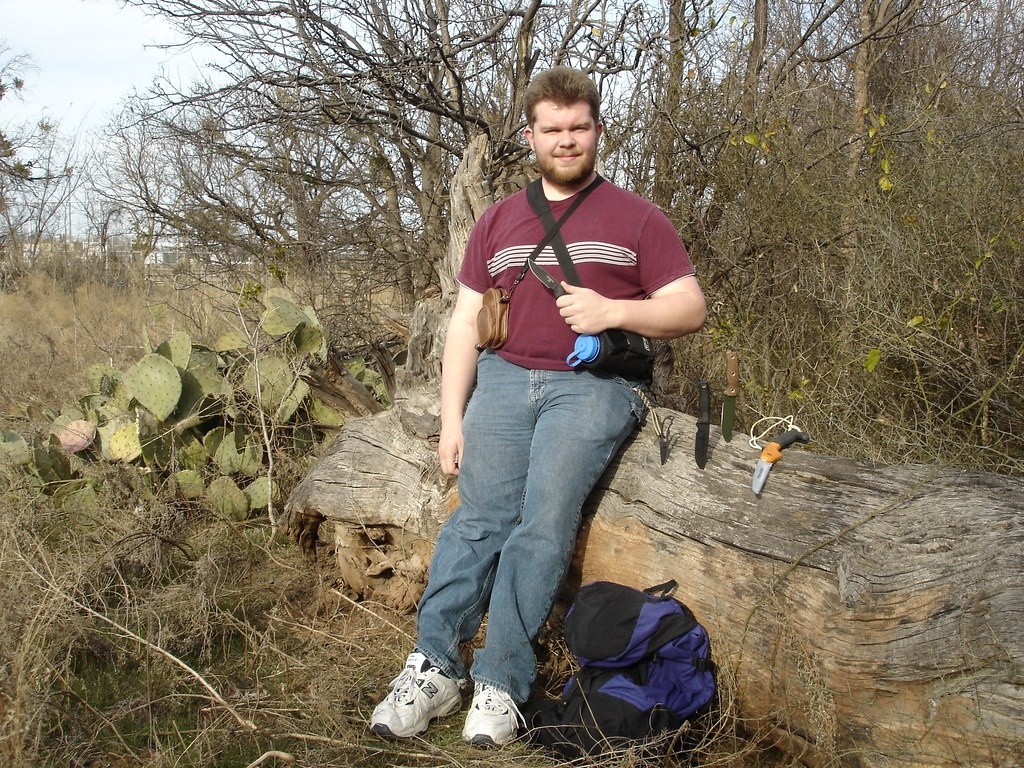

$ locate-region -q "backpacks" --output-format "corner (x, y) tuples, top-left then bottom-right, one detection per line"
(515, 580), (716, 768)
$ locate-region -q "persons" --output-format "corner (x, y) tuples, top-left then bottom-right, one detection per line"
(373, 68), (711, 743)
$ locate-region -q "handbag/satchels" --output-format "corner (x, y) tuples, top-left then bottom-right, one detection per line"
(477, 286), (509, 350)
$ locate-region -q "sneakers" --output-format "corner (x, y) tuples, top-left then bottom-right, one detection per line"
(371, 653), (528, 749)
